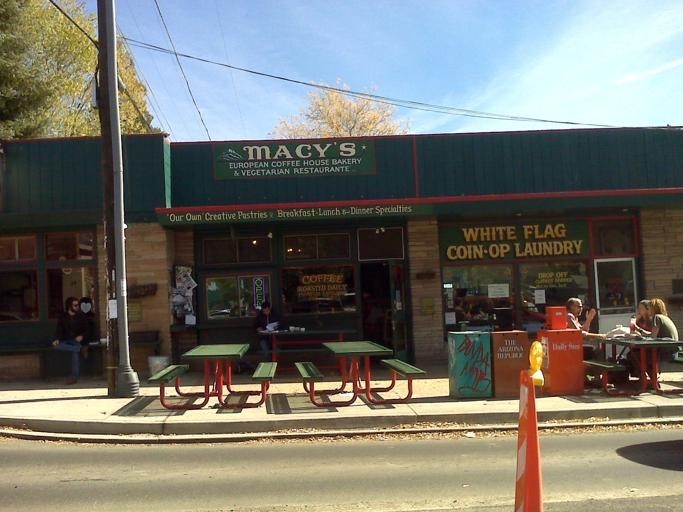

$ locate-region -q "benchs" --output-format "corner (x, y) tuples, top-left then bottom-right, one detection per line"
(148, 360), (428, 409)
(1, 321), (107, 356)
(582, 357), (682, 397)
(254, 349), (351, 374)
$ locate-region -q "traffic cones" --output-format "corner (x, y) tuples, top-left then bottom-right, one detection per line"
(512, 370), (544, 512)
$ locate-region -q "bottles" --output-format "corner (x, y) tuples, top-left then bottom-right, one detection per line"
(630, 315), (636, 334)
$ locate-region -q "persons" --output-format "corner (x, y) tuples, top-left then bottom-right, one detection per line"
(618, 300), (654, 336)
(566, 298), (604, 388)
(567, 262), (594, 298)
(455, 282), (539, 321)
(627, 298), (678, 390)
(41, 298), (89, 385)
(253, 301), (279, 361)
(80, 296), (97, 317)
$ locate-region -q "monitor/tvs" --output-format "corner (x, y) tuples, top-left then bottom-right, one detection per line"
(202, 237), (237, 266)
(237, 236), (272, 264)
(283, 234), (317, 261)
(317, 233), (351, 260)
(357, 227), (405, 262)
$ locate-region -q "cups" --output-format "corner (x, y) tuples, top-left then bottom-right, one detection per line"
(615, 324), (622, 333)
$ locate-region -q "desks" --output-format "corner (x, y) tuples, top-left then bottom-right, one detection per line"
(254, 328), (357, 371)
(587, 323), (682, 396)
(302, 340), (413, 408)
(160, 343), (269, 409)
